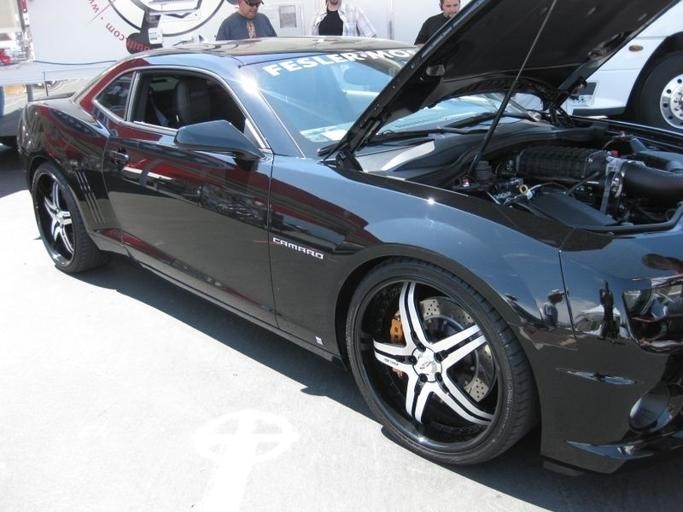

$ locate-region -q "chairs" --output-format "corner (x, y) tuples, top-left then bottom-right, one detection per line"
(171, 77), (213, 129)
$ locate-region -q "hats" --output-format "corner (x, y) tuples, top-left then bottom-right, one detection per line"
(245, 0), (264, 5)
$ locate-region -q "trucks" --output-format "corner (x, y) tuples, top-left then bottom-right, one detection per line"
(1, 1), (682, 148)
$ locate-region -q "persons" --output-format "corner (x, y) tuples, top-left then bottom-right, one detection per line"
(310, 0), (377, 38)
(540, 289), (565, 325)
(642, 253), (683, 276)
(599, 281), (614, 321)
(216, 0), (277, 40)
(652, 297), (666, 318)
(414, 0), (460, 44)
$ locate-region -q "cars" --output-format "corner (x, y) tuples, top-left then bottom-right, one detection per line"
(17, 1), (682, 493)
